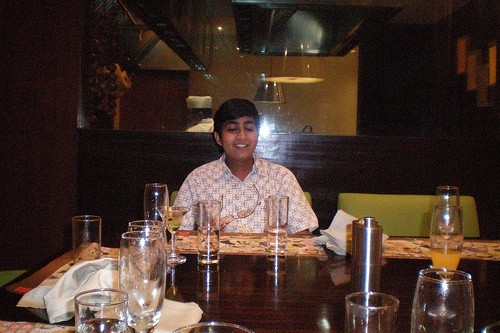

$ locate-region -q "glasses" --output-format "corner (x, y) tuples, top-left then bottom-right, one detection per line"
(218, 185), (258, 227)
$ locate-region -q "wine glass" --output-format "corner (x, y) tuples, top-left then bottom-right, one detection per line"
(428, 205), (464, 319)
(434, 186), (459, 259)
(127, 220), (163, 301)
(157, 203), (190, 261)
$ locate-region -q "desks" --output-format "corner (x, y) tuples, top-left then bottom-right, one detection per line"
(0, 229), (500, 333)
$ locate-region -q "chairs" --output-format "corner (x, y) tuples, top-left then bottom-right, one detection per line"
(338, 193), (480, 238)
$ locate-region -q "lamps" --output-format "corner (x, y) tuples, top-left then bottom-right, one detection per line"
(253, 8), (285, 104)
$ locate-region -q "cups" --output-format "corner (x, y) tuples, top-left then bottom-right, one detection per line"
(74, 288), (130, 333)
(172, 321), (256, 333)
(267, 254), (287, 278)
(71, 215), (103, 266)
(197, 200), (221, 265)
(344, 291), (400, 333)
(265, 195), (289, 254)
(197, 265), (220, 312)
(352, 217), (382, 268)
(119, 231), (167, 333)
(143, 183), (170, 227)
(410, 268), (475, 333)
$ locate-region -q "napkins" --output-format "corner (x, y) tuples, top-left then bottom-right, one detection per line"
(311, 209), (389, 256)
(16, 257), (203, 333)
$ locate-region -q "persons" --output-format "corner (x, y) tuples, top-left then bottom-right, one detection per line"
(182, 96), (215, 133)
(170, 98), (319, 234)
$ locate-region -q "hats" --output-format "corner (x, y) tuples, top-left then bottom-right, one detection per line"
(186, 96), (212, 109)
